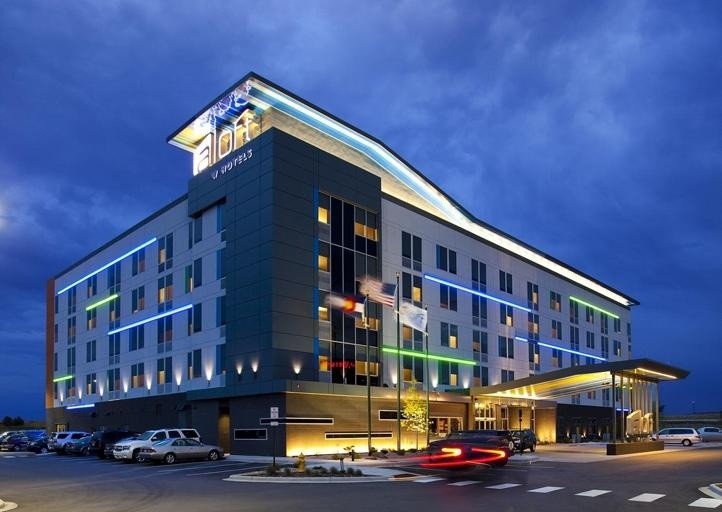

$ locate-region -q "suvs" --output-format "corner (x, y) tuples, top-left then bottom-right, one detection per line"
(113, 428), (202, 463)
(426, 428), (537, 471)
(0, 430), (224, 463)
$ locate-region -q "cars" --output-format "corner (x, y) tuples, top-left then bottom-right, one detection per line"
(697, 427), (722, 443)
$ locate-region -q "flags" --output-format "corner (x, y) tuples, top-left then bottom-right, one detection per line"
(359, 274), (397, 308)
(397, 302), (428, 333)
(327, 292), (367, 316)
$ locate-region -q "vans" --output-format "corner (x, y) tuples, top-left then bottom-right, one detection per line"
(648, 428), (703, 446)
